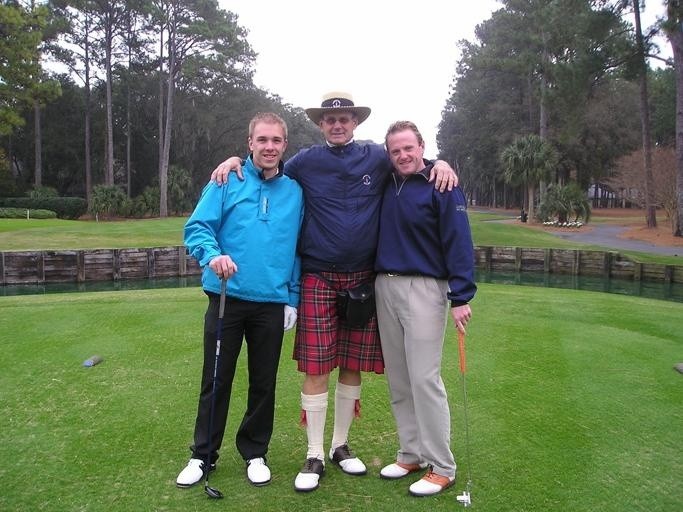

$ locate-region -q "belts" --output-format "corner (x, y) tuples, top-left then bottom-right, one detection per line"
(384, 271), (422, 276)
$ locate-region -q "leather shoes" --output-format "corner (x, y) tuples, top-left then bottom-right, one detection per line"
(176, 458), (216, 488)
(329, 444), (366, 474)
(409, 468), (455, 496)
(295, 458), (325, 490)
(246, 457), (271, 486)
(380, 461), (428, 478)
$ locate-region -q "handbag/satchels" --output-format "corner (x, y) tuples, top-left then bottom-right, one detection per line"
(335, 280), (376, 324)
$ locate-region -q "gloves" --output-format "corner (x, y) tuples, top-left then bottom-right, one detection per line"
(284, 305), (297, 330)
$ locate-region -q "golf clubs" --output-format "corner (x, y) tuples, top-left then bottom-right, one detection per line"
(456, 327), (471, 506)
(203, 277), (228, 500)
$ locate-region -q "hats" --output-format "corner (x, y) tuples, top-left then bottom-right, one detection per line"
(305, 92), (371, 125)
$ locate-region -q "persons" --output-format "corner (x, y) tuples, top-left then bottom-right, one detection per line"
(171, 112), (310, 492)
(207, 91), (460, 495)
(362, 119), (480, 499)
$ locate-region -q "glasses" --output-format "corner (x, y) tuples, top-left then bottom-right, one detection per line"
(322, 116), (354, 125)
